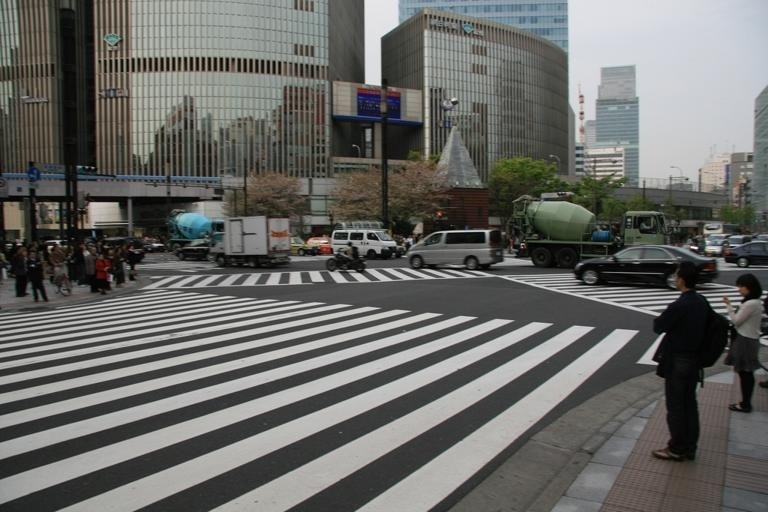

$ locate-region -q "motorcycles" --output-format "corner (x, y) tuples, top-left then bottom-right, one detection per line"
(326, 247), (366, 273)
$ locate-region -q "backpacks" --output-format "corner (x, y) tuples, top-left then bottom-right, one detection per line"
(695, 292), (729, 369)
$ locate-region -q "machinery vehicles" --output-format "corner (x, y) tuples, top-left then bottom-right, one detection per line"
(164, 207), (224, 252)
(510, 190), (678, 270)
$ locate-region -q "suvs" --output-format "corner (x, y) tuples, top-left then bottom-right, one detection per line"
(145, 237), (163, 252)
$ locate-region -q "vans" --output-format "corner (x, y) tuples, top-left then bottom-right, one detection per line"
(331, 229), (397, 259)
(404, 230), (505, 269)
(687, 223), (768, 255)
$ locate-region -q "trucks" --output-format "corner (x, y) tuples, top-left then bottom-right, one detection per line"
(206, 212), (293, 267)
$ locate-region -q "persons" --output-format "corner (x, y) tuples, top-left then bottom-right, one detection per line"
(722, 272), (762, 413)
(393, 234), (426, 251)
(0, 238), (137, 302)
(336, 242), (355, 267)
(652, 259), (708, 462)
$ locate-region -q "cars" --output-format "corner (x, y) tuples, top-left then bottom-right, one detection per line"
(45, 239), (67, 252)
(290, 235), (318, 257)
(574, 244), (717, 290)
(170, 237), (222, 260)
(397, 245), (406, 258)
(724, 240), (768, 267)
(307, 236), (333, 254)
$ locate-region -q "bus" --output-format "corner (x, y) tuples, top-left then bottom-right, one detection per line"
(100, 237), (146, 265)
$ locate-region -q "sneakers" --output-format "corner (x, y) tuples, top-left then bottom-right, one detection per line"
(728, 403), (752, 413)
(652, 446), (696, 462)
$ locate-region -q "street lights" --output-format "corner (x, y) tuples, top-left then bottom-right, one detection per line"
(548, 154), (561, 175)
(591, 158), (618, 212)
(351, 143), (361, 156)
(668, 176), (689, 198)
(378, 77), (391, 227)
